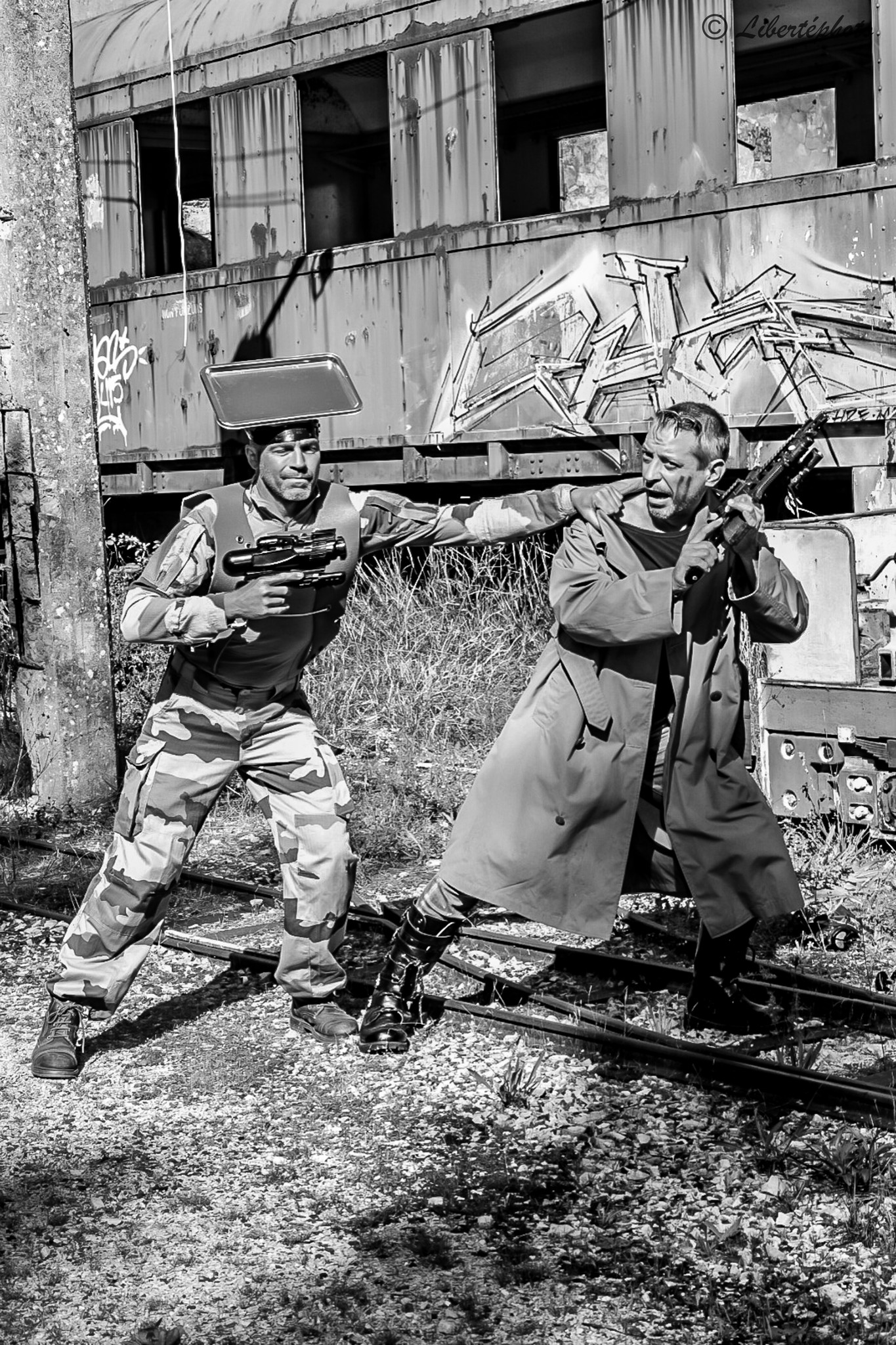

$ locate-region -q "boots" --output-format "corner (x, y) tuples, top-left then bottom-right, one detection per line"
(683, 911), (786, 1034)
(358, 896), (460, 1052)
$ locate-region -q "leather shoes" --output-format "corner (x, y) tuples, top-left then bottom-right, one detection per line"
(31, 994), (85, 1078)
(290, 990), (359, 1044)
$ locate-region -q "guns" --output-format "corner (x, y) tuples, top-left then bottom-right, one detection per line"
(221, 527), (349, 592)
(679, 411), (831, 645)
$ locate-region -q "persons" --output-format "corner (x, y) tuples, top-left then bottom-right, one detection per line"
(352, 400), (810, 1054)
(27, 417), (623, 1081)
